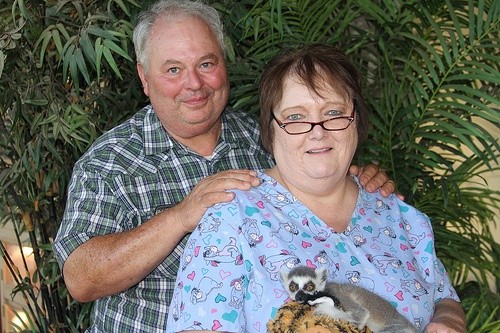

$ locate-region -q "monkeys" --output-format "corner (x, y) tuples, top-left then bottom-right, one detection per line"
(278, 267), (418, 333)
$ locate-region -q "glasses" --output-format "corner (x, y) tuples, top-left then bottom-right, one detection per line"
(268, 101), (356, 135)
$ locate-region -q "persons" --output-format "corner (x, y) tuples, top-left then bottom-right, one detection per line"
(51, 0), (404, 333)
(167, 46), (466, 333)
